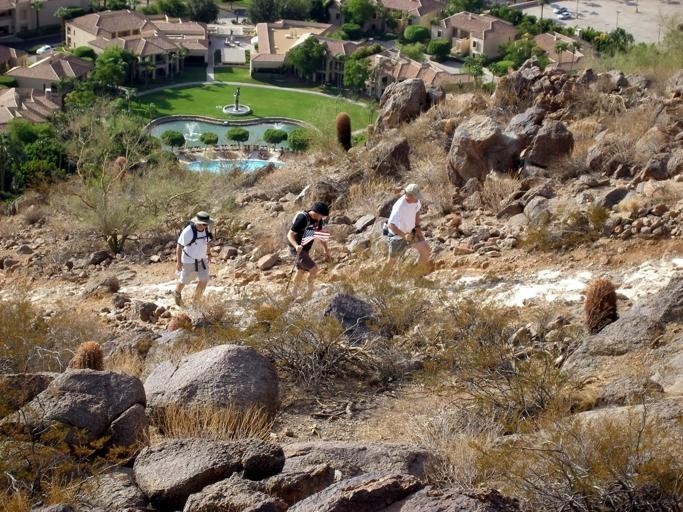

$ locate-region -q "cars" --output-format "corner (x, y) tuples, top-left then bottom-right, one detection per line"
(35, 44), (52, 54)
(553, 7), (566, 14)
(559, 12), (570, 20)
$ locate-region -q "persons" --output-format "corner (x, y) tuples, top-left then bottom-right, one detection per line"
(173, 211), (215, 308)
(380, 183), (429, 278)
(279, 148), (284, 157)
(287, 201), (330, 296)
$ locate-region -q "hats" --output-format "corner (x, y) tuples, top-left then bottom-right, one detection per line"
(405, 183), (424, 200)
(191, 211), (214, 225)
(311, 203), (329, 216)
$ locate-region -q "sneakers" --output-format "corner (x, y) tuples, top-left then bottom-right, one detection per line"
(173, 290), (184, 307)
